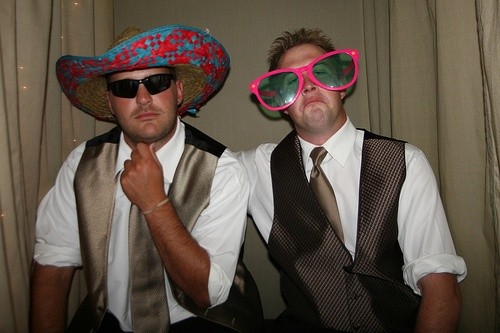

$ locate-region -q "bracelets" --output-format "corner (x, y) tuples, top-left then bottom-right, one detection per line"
(141, 196), (170, 217)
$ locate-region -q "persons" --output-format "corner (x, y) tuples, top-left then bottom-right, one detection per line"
(25, 26), (267, 333)
(222, 26), (468, 333)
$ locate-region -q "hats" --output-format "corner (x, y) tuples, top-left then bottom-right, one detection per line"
(55, 25), (231, 126)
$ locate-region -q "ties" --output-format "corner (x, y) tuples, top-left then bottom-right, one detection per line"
(309, 147), (345, 246)
(128, 203), (171, 333)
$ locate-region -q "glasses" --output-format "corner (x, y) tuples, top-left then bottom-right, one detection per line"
(250, 49), (362, 111)
(106, 73), (174, 98)
